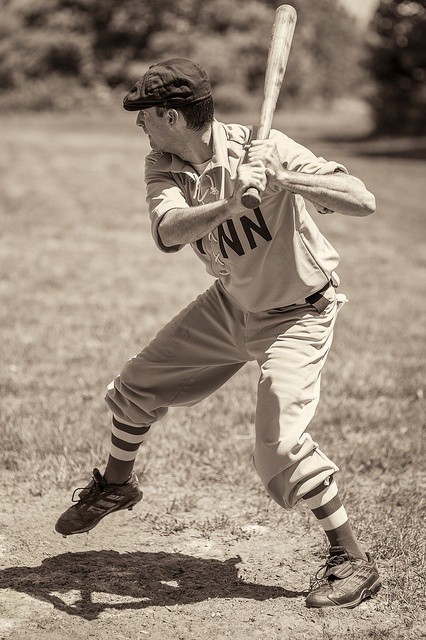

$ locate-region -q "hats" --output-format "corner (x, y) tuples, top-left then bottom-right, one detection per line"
(124, 59), (210, 110)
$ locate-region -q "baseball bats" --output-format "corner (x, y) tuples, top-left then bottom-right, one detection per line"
(240, 5), (297, 209)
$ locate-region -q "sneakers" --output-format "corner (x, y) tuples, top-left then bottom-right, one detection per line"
(306, 547), (382, 609)
(55, 469), (143, 536)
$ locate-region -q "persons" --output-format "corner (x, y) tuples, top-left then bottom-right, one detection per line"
(54, 58), (382, 609)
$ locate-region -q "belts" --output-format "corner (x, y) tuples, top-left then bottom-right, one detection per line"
(306, 284), (331, 308)
